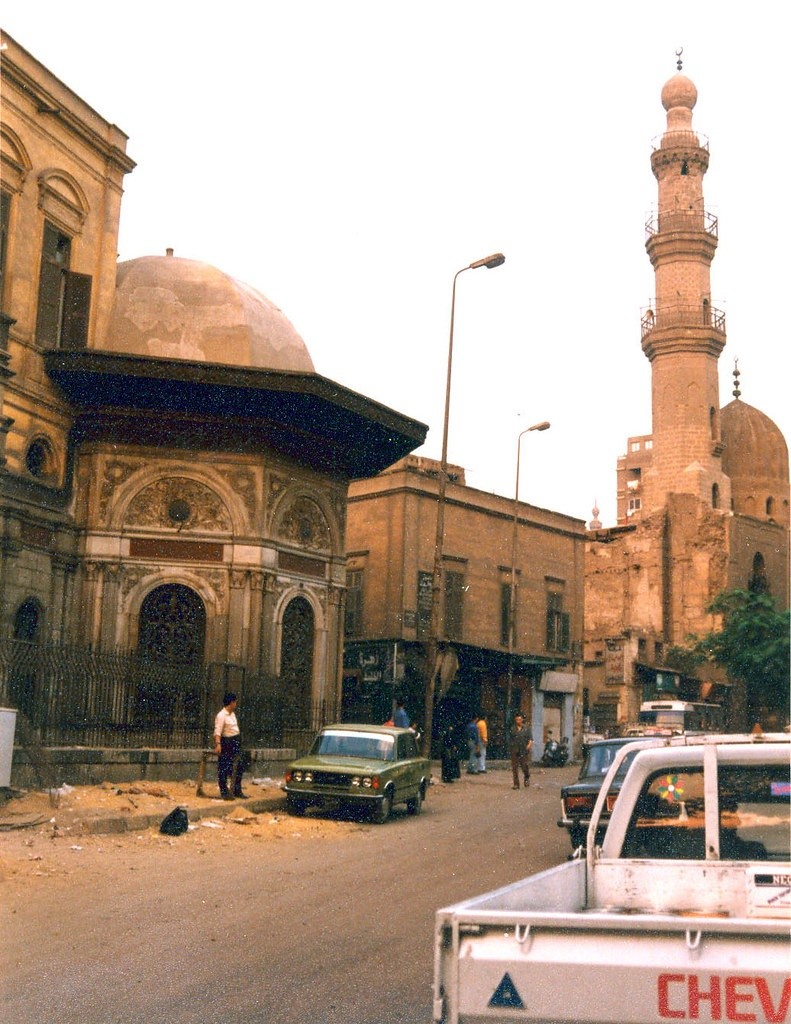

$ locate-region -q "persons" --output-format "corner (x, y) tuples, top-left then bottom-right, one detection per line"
(214, 693), (249, 800)
(383, 702), (416, 734)
(510, 714), (533, 790)
(442, 714), (488, 782)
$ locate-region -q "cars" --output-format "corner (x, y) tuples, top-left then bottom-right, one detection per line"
(557, 737), (739, 854)
(280, 724), (431, 819)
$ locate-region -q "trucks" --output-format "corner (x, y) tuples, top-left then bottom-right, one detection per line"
(427, 729), (790, 1024)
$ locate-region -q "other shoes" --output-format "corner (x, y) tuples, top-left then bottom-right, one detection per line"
(443, 779), (454, 783)
(524, 776), (530, 787)
(512, 781), (519, 789)
(479, 770), (487, 773)
(466, 770), (479, 775)
(220, 790), (235, 800)
(232, 789), (249, 799)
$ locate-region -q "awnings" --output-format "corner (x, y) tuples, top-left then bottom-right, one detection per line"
(635, 661), (703, 682)
(436, 638), (579, 667)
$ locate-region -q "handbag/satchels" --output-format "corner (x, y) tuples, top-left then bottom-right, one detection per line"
(160, 807), (189, 837)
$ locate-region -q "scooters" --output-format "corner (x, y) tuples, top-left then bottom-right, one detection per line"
(541, 729), (569, 769)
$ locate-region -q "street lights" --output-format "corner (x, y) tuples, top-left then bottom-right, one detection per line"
(502, 419), (553, 716)
(423, 250), (506, 786)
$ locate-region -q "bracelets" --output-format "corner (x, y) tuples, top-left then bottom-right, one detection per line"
(216, 742), (221, 744)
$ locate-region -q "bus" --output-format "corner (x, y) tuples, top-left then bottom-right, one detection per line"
(635, 701), (725, 742)
